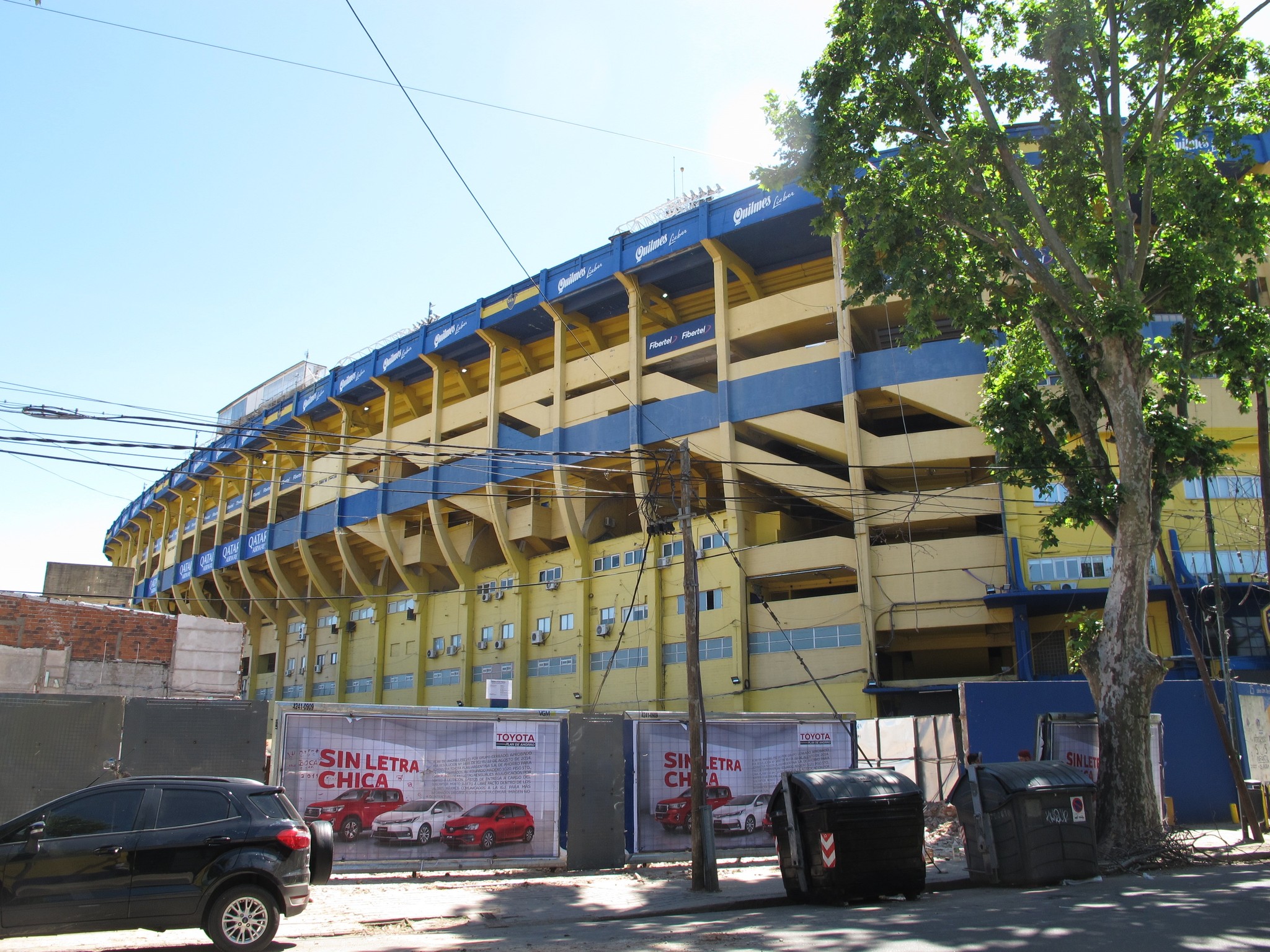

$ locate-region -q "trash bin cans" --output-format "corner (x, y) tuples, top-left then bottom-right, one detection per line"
(944, 760), (1099, 888)
(765, 771), (926, 907)
(1241, 779), (1266, 824)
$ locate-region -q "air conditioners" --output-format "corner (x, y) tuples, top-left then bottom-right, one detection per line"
(299, 668), (304, 674)
(284, 670), (291, 676)
(1032, 583), (1051, 591)
(314, 665), (321, 672)
(1060, 582), (1077, 590)
(299, 627), (305, 633)
(482, 593), (490, 602)
(427, 649), (436, 658)
(495, 591), (502, 599)
(447, 645), (457, 656)
(477, 641), (485, 649)
(299, 633), (306, 639)
(494, 640), (504, 649)
(531, 629), (543, 643)
(546, 580), (557, 589)
(694, 548), (705, 559)
(596, 624), (609, 635)
(656, 556), (669, 568)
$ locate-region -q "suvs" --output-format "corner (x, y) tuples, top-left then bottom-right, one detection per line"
(1, 773), (335, 951)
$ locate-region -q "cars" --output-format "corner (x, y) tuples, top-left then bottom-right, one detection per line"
(371, 799), (534, 850)
(712, 793), (772, 836)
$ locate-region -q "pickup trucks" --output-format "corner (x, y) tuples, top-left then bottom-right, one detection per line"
(656, 783), (737, 832)
(305, 786), (413, 842)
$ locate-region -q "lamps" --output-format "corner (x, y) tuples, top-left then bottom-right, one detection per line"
(731, 676), (740, 684)
(1000, 584), (1011, 594)
(985, 584), (996, 595)
